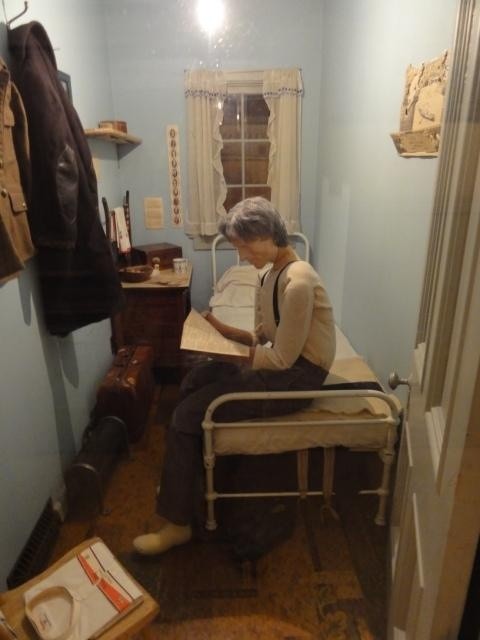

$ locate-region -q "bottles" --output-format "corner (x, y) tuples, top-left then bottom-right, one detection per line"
(151, 264), (160, 283)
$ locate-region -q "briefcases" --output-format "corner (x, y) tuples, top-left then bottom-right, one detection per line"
(96, 345), (155, 442)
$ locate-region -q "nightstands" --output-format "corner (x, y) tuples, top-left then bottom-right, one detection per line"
(110, 258), (197, 388)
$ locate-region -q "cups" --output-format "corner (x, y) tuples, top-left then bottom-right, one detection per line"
(172, 257), (189, 278)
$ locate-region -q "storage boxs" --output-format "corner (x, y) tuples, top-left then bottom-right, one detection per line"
(130, 241), (184, 272)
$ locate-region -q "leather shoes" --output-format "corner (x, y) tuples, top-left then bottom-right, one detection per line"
(134, 520), (192, 557)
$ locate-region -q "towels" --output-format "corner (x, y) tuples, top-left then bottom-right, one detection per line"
(109, 205), (134, 256)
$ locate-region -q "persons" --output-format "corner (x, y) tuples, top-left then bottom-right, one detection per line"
(133, 196), (336, 556)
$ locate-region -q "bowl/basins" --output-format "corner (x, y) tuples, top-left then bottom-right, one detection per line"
(99, 121), (128, 133)
(119, 265), (153, 283)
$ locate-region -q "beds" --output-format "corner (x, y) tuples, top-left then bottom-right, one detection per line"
(197, 229), (407, 537)
